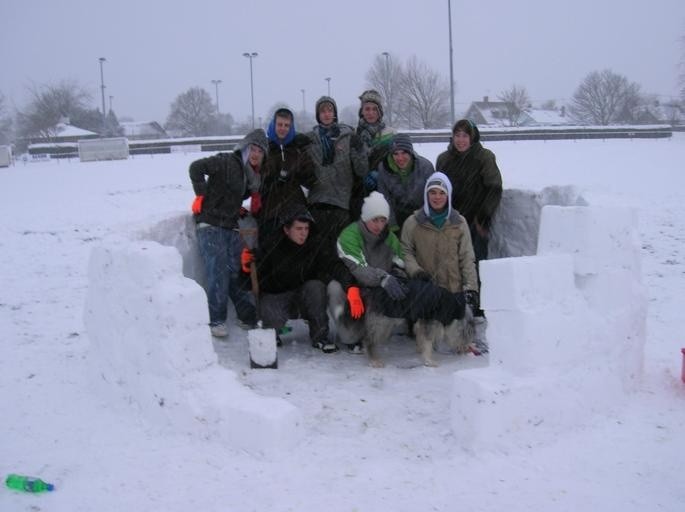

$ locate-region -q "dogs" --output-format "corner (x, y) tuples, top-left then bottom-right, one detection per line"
(334, 278), (489, 369)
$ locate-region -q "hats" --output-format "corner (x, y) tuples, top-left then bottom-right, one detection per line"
(360, 190), (392, 224)
(388, 133), (415, 158)
(426, 178), (449, 196)
(357, 89), (385, 121)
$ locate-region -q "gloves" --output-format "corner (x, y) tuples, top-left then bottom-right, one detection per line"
(346, 285), (366, 319)
(463, 289), (480, 309)
(381, 272), (410, 301)
(191, 195), (205, 215)
(240, 247), (255, 274)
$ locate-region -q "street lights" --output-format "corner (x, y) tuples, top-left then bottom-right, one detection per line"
(323, 76), (331, 97)
(381, 50), (392, 128)
(98, 55), (107, 138)
(211, 79), (222, 114)
(240, 51), (260, 131)
(298, 89), (307, 133)
(107, 95), (113, 110)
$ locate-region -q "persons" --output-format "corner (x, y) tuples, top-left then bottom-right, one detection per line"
(188, 128), (269, 338)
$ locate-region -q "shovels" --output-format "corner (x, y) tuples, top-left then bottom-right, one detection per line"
(237, 226), (279, 367)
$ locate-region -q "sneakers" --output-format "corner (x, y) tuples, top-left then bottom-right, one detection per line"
(312, 339), (337, 353)
(346, 342), (365, 354)
(210, 320), (229, 337)
